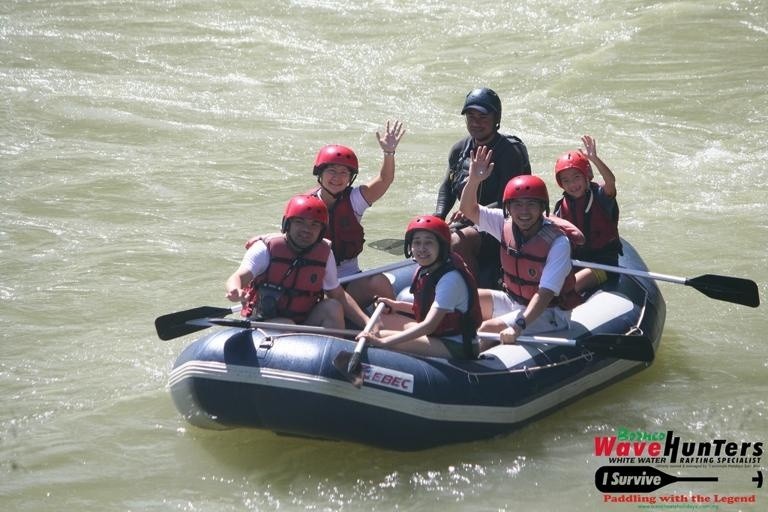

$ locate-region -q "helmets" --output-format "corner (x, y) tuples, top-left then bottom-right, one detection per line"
(464, 87), (502, 121)
(503, 175), (549, 210)
(280, 193), (330, 234)
(554, 150), (594, 188)
(405, 215), (451, 248)
(312, 144), (359, 176)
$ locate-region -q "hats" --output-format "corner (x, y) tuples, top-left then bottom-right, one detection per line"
(460, 103), (490, 116)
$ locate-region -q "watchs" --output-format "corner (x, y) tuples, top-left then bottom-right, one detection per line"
(515, 316), (527, 330)
(383, 151), (395, 156)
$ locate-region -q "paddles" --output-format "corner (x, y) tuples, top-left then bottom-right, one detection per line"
(476, 331), (655, 362)
(186, 315), (365, 346)
(572, 259), (760, 308)
(332, 295), (391, 388)
(367, 201), (500, 256)
(155, 256), (418, 342)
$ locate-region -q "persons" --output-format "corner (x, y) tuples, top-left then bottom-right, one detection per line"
(434, 88), (532, 288)
(357, 216), (477, 361)
(554, 136), (623, 292)
(225, 193), (372, 336)
(304, 120), (405, 280)
(459, 146), (586, 352)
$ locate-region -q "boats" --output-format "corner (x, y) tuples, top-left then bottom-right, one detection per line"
(168, 236), (666, 453)
